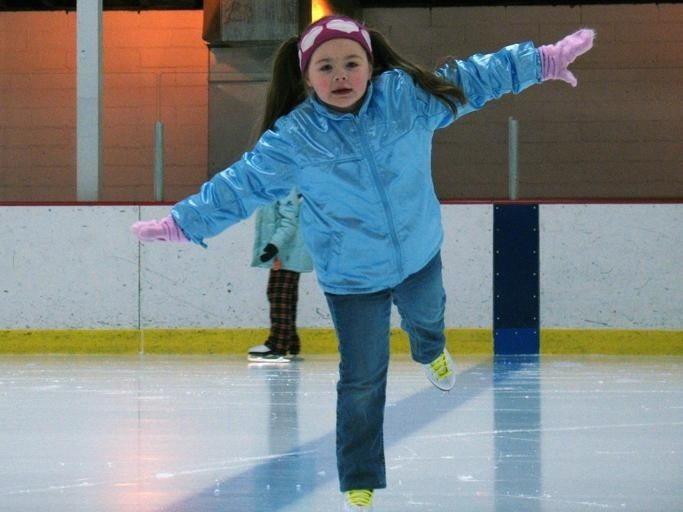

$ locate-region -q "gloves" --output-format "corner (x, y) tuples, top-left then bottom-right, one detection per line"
(540, 29), (594, 87)
(129, 214), (188, 244)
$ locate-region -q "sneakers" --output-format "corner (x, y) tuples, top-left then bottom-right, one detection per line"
(247, 345), (297, 359)
(347, 489), (374, 512)
(423, 347), (458, 391)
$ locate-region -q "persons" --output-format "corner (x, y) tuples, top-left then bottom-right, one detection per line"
(131, 11), (596, 510)
(245, 189), (313, 360)
